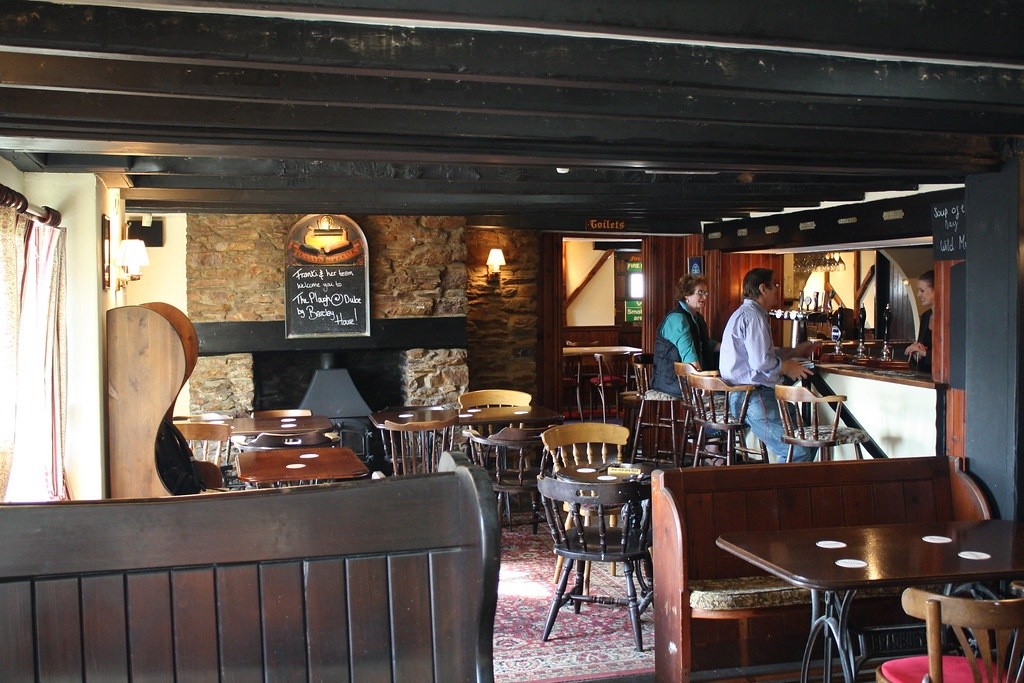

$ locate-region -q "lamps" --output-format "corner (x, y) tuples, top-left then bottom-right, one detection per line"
(142, 212), (152, 226)
(116, 240), (150, 280)
(556, 167), (569, 173)
(486, 248), (506, 282)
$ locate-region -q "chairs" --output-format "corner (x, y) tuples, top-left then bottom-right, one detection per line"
(167, 304), (1024, 683)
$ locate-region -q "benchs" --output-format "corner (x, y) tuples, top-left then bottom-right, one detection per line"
(650, 454), (992, 683)
(0, 466), (502, 683)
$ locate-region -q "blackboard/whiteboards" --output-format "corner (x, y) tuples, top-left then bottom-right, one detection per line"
(284, 214), (370, 339)
(930, 200), (967, 261)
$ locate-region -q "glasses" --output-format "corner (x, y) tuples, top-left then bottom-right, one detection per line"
(758, 282), (779, 288)
(692, 291), (708, 296)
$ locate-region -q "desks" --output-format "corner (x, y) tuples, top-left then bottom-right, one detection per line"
(800, 311), (824, 337)
(563, 346), (642, 422)
(821, 351), (930, 377)
(367, 408), (461, 476)
(453, 405), (566, 470)
(224, 416), (333, 448)
(171, 419), (229, 464)
(807, 337), (913, 351)
(716, 519), (1024, 683)
(234, 446), (369, 489)
(555, 464), (659, 604)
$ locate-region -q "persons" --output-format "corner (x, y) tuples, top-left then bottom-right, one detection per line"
(719, 266), (818, 463)
(904, 270), (935, 373)
(650, 273), (723, 466)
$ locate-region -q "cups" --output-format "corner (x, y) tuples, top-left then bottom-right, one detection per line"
(810, 339), (823, 362)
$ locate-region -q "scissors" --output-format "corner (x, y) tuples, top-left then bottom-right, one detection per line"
(597, 463), (619, 473)
